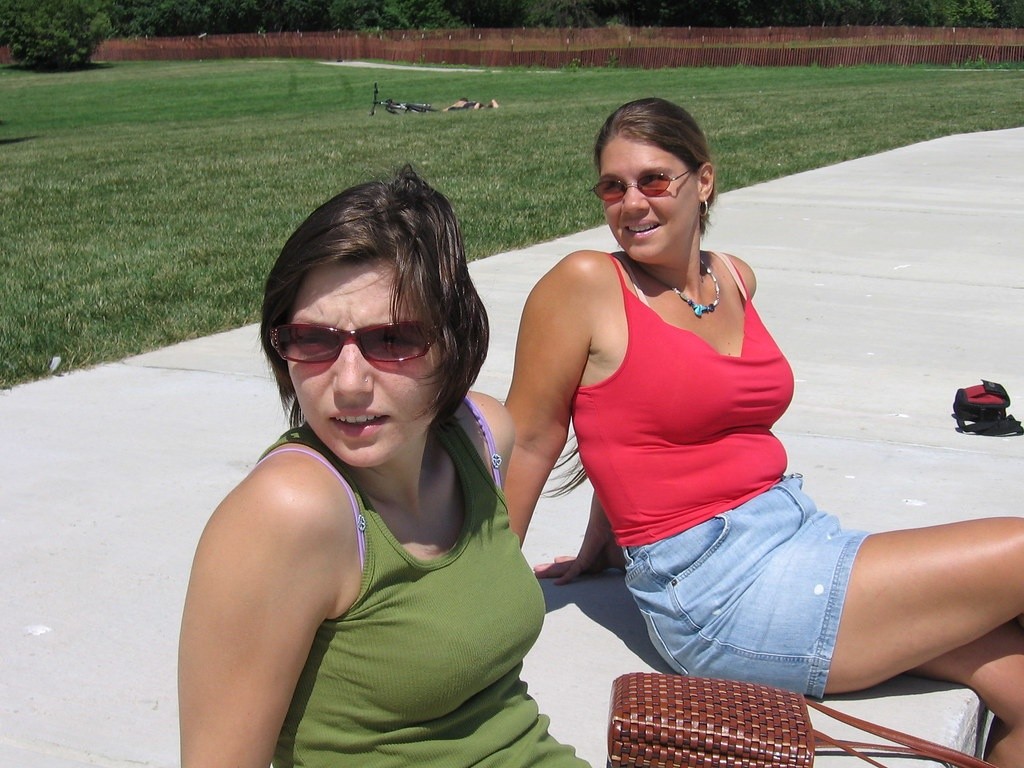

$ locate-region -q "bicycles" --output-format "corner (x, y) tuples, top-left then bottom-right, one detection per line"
(368, 82), (438, 115)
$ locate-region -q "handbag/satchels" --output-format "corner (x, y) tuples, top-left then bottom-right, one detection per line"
(607, 671), (997, 768)
(953, 378), (1024, 436)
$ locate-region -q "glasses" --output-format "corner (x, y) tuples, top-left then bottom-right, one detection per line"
(589, 161), (703, 203)
(269, 313), (442, 364)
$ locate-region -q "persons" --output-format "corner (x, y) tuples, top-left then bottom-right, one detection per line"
(178, 162), (589, 768)
(501, 96), (1024, 768)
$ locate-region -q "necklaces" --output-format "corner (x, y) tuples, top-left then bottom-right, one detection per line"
(672, 259), (721, 316)
